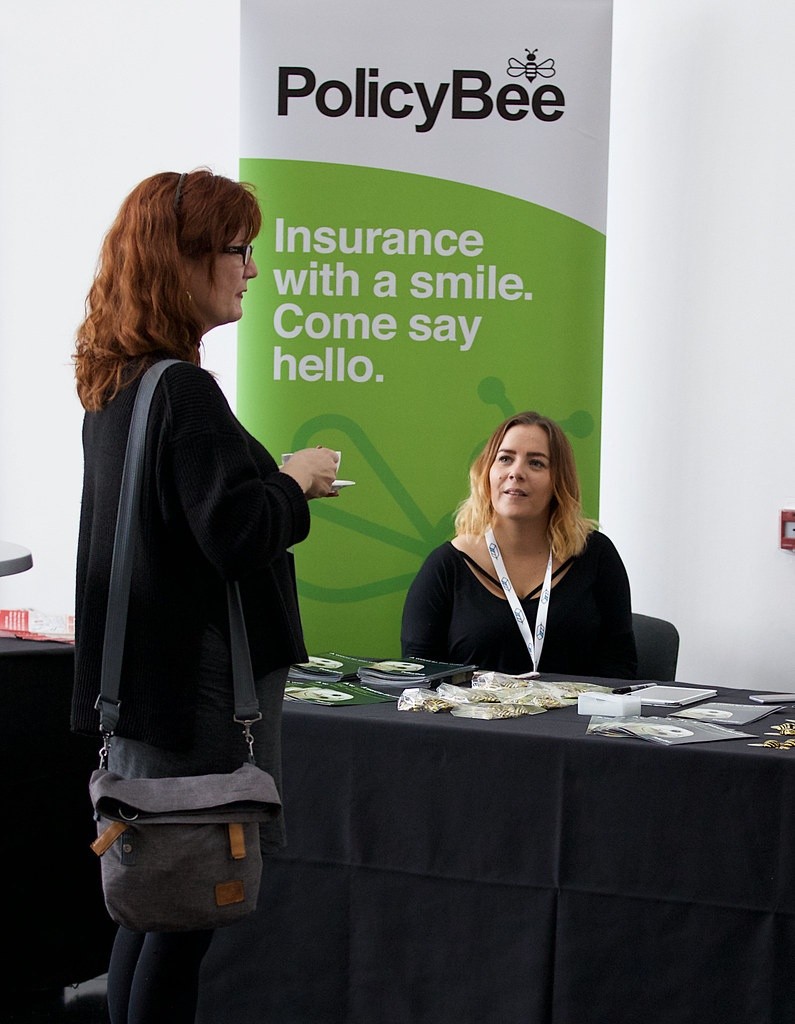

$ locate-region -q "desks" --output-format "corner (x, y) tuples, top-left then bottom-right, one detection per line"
(195, 670), (795, 1024)
(0, 636), (105, 1024)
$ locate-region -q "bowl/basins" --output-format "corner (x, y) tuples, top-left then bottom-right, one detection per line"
(281, 451), (342, 476)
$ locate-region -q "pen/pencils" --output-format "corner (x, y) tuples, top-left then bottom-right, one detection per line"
(612, 682), (657, 694)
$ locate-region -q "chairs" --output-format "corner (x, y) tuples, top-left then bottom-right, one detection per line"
(627, 612), (679, 684)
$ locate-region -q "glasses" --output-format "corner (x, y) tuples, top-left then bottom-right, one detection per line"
(183, 245), (253, 265)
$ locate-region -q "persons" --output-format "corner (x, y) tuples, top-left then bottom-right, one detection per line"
(75, 171), (345, 1024)
(400, 411), (639, 681)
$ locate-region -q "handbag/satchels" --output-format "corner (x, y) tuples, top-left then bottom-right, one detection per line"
(89, 764), (283, 928)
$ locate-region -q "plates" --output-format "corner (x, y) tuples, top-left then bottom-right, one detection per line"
(331, 480), (356, 491)
